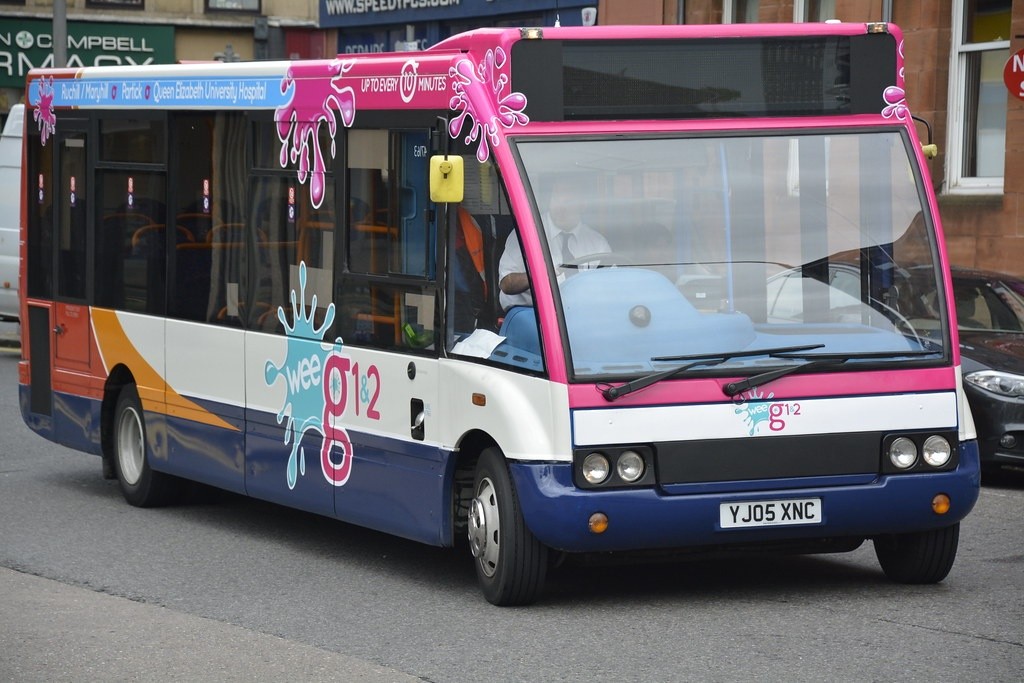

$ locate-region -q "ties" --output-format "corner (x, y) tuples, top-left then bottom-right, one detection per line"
(558, 231), (580, 280)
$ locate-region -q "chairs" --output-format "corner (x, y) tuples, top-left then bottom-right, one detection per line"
(40, 192), (395, 349)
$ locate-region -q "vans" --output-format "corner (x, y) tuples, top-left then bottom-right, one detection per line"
(1, 104), (23, 353)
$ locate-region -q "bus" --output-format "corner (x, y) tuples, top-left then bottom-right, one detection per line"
(16, 23), (977, 606)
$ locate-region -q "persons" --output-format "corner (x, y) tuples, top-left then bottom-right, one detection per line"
(496, 172), (620, 316)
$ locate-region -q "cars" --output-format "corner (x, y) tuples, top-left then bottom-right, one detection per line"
(680, 257), (1024, 479)
